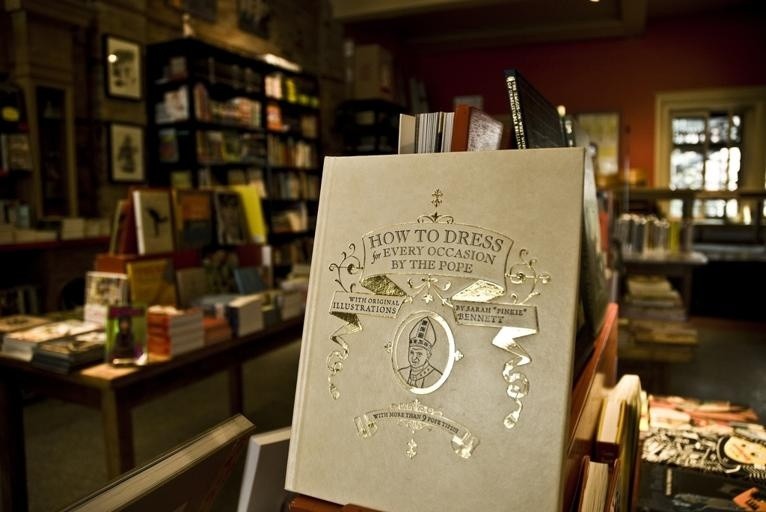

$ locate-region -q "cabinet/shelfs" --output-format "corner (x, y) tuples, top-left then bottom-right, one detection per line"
(0, 0), (321, 312)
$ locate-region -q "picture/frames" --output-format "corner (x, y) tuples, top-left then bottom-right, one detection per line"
(103, 32), (146, 184)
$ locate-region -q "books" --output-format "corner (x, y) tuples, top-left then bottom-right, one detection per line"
(284, 146), (586, 511)
(62, 412), (256, 511)
(236, 426), (296, 511)
(558, 107), (704, 376)
(398, 68), (566, 155)
(1, 33), (321, 377)
(577, 374), (766, 511)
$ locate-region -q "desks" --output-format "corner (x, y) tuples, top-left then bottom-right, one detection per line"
(0, 305), (306, 512)
